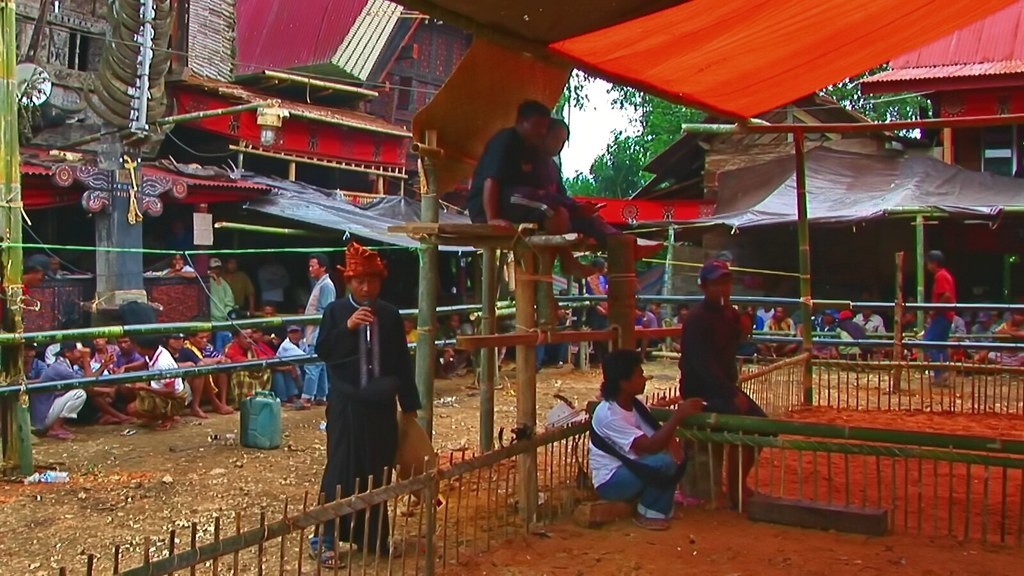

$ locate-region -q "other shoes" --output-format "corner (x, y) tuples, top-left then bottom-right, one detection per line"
(556, 364), (563, 369)
(672, 507), (685, 520)
(631, 512), (671, 530)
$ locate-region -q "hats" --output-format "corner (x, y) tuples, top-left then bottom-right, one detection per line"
(24, 342), (38, 349)
(821, 308), (837, 316)
(839, 310), (853, 320)
(52, 339), (84, 356)
(700, 258), (731, 282)
(208, 257), (223, 268)
(287, 324), (302, 332)
(343, 241), (389, 280)
(392, 410), (439, 503)
(974, 310), (991, 322)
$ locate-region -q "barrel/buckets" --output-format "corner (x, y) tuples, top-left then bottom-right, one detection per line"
(241, 391), (280, 450)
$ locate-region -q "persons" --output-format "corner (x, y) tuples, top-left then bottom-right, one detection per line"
(678, 259), (780, 513)
(21, 252), (1023, 442)
(922, 250), (961, 387)
(308, 241), (421, 569)
(469, 98), (660, 274)
(588, 347), (704, 531)
(292, 253), (337, 411)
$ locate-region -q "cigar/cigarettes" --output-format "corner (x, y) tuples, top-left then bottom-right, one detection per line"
(702, 401), (708, 406)
(720, 296), (724, 306)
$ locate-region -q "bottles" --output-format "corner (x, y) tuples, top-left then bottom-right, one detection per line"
(39, 471), (69, 483)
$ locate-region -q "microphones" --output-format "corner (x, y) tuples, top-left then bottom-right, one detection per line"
(362, 301), (372, 349)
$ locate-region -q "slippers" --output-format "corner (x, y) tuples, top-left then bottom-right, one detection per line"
(281, 399), (327, 410)
(47, 431), (76, 439)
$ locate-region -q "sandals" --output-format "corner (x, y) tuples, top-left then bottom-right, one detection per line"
(357, 536), (401, 558)
(308, 536), (345, 569)
